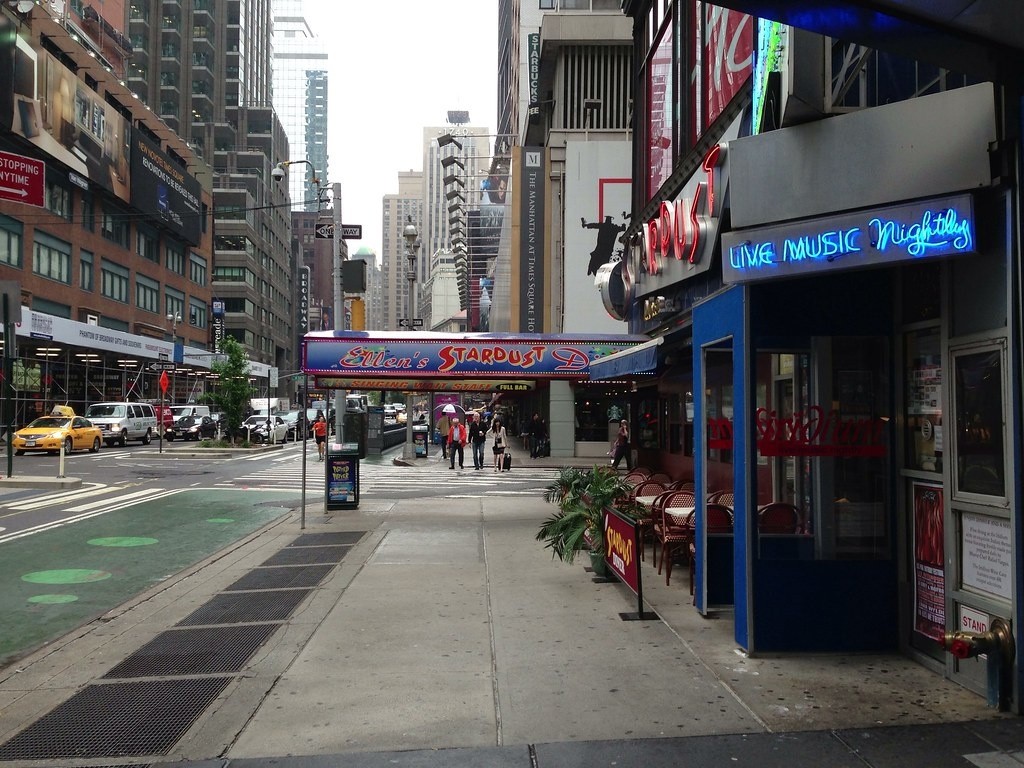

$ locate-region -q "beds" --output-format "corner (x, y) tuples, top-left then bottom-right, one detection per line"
(12, 93), (89, 180)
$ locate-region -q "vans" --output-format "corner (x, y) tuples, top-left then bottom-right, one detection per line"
(77, 400), (158, 448)
(169, 404), (210, 421)
(151, 405), (175, 442)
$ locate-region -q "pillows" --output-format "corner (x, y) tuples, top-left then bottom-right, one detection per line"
(17, 100), (40, 139)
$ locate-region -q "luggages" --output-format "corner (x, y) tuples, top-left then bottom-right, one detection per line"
(498, 447), (511, 471)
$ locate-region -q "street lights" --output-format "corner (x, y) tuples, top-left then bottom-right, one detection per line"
(301, 264), (311, 333)
(271, 159), (345, 444)
(402, 224), (421, 460)
(166, 311), (183, 407)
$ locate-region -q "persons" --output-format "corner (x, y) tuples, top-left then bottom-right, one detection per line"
(480, 416), (492, 430)
(520, 412), (549, 460)
(448, 418), (467, 469)
(468, 412), (488, 470)
(312, 416), (327, 461)
(612, 420), (632, 471)
(323, 313), (332, 330)
(436, 412), (452, 459)
(486, 419), (508, 472)
(481, 176), (509, 203)
(495, 410), (509, 435)
(415, 405), (420, 416)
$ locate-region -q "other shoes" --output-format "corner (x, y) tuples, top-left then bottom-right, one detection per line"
(495, 469), (497, 472)
(449, 466), (454, 469)
(500, 469), (504, 472)
(461, 465), (464, 469)
(475, 468), (479, 470)
(480, 464), (483, 469)
(319, 456), (321, 460)
(321, 457), (325, 461)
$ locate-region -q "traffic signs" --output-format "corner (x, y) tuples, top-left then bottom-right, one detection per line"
(149, 362), (177, 372)
(398, 318), (424, 327)
(314, 222), (363, 240)
(0, 150), (48, 211)
(158, 352), (168, 363)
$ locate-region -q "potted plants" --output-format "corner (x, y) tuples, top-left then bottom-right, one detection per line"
(535, 464), (651, 578)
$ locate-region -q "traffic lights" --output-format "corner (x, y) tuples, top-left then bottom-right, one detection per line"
(341, 258), (368, 294)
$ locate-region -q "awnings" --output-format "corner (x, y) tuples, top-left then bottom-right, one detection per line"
(589, 337), (663, 380)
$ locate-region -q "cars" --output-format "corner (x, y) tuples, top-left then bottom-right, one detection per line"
(383, 404), (397, 420)
(10, 404), (104, 457)
(241, 414), (289, 443)
(392, 401), (408, 423)
(273, 409), (311, 440)
(209, 392), (384, 438)
(165, 416), (218, 442)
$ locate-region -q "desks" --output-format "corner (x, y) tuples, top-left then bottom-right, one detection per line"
(635, 495), (662, 506)
(665, 505), (767, 519)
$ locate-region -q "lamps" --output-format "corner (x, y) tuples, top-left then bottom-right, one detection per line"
(443, 174), (511, 188)
(40, 34), (79, 44)
(582, 99), (601, 141)
(109, 93), (138, 99)
(134, 118), (164, 124)
(122, 104), (151, 111)
(441, 155), (512, 170)
(626, 99), (634, 141)
(448, 203), (511, 312)
(58, 50), (95, 61)
(20, 16), (60, 29)
(446, 189), (512, 203)
(75, 65), (111, 73)
(147, 128), (219, 176)
(94, 80), (126, 87)
(436, 133), (519, 150)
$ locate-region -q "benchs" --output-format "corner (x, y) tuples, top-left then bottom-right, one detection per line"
(72, 132), (103, 166)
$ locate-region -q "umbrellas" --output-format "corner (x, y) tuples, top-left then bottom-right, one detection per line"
(434, 403), (465, 413)
(484, 412), (492, 415)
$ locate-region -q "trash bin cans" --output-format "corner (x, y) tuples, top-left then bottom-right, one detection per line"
(413, 424), (428, 458)
(326, 441), (359, 511)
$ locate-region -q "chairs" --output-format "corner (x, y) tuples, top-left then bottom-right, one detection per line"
(594, 466), (806, 606)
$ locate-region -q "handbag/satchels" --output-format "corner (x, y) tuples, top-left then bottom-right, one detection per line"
(609, 445), (619, 459)
